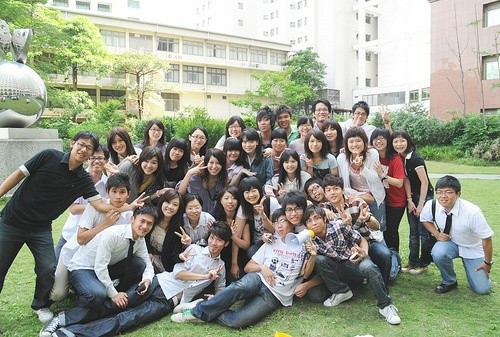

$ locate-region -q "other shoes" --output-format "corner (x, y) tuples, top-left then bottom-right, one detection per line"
(401, 264), (415, 272)
(409, 266), (428, 274)
(33, 308), (54, 322)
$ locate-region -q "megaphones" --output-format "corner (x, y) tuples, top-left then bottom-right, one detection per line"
(285, 230), (315, 251)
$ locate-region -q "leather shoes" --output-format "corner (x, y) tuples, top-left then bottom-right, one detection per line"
(435, 281), (458, 294)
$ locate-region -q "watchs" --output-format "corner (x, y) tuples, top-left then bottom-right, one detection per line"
(382, 175), (388, 180)
(484, 261), (493, 265)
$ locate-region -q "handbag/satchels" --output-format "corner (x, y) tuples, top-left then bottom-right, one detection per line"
(422, 237), (437, 263)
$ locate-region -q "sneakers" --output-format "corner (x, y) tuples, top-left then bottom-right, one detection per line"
(173, 298), (204, 313)
(171, 310), (205, 323)
(379, 305), (401, 325)
(40, 317), (59, 337)
(324, 290), (353, 307)
(52, 330), (68, 337)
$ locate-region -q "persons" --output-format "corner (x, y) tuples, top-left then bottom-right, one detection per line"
(303, 206), (401, 325)
(172, 209), (317, 328)
(52, 99), (407, 301)
(68, 205), (158, 308)
(40, 222), (231, 337)
(391, 131), (434, 274)
(0, 131), (150, 323)
(52, 173), (133, 303)
(419, 176), (494, 294)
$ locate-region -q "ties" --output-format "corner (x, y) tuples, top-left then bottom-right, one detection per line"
(126, 240), (135, 290)
(443, 212), (453, 234)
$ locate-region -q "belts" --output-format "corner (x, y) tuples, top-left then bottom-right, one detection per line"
(172, 296), (178, 308)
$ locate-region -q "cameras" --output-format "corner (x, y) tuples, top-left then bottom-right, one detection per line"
(349, 253), (358, 261)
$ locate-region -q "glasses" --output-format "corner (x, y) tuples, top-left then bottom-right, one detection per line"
(186, 206), (201, 212)
(89, 155), (105, 163)
(75, 140), (94, 152)
(228, 124), (241, 129)
(150, 128), (162, 133)
(316, 109), (327, 112)
(272, 185), (321, 228)
(373, 137), (384, 141)
(190, 135), (206, 141)
(355, 111), (367, 116)
(436, 190), (454, 198)
